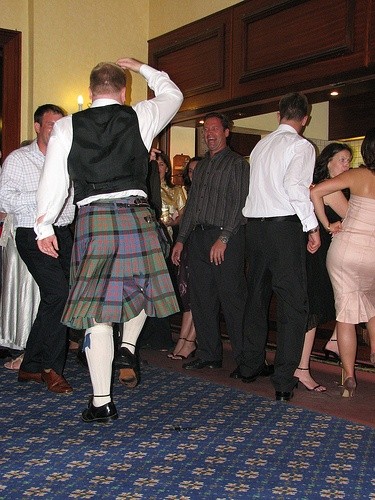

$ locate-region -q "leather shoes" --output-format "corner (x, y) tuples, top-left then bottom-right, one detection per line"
(114, 342), (138, 367)
(81, 395), (119, 422)
(229, 365), (241, 379)
(182, 359), (207, 369)
(40, 368), (74, 396)
(241, 364), (275, 383)
(205, 361), (223, 369)
(18, 366), (42, 382)
(275, 377), (299, 400)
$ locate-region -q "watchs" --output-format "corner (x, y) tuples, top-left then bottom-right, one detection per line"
(221, 234), (231, 246)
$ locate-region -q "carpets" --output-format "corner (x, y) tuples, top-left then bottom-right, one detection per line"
(0, 350), (375, 500)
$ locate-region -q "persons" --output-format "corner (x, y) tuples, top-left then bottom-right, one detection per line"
(170, 113), (256, 380)
(243, 97), (321, 407)
(142, 138), (215, 364)
(0, 107), (97, 394)
(27, 56), (190, 425)
(306, 133), (374, 400)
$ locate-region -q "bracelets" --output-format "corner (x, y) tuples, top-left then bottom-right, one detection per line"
(307, 226), (323, 238)
(327, 220), (333, 234)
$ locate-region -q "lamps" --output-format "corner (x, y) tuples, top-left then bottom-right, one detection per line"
(329, 88), (343, 96)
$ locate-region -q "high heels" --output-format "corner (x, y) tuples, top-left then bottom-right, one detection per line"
(323, 340), (343, 362)
(293, 368), (326, 393)
(172, 340), (197, 359)
(168, 338), (187, 357)
(338, 376), (356, 397)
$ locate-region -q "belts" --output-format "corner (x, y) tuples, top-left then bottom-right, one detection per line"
(248, 215), (301, 222)
(194, 223), (225, 232)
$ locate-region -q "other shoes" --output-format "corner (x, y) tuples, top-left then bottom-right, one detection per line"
(120, 368), (138, 387)
(69, 341), (79, 349)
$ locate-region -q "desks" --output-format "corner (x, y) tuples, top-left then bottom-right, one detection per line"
(0, 237), (41, 350)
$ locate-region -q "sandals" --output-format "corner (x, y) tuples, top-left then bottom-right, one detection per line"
(4, 353), (25, 370)
